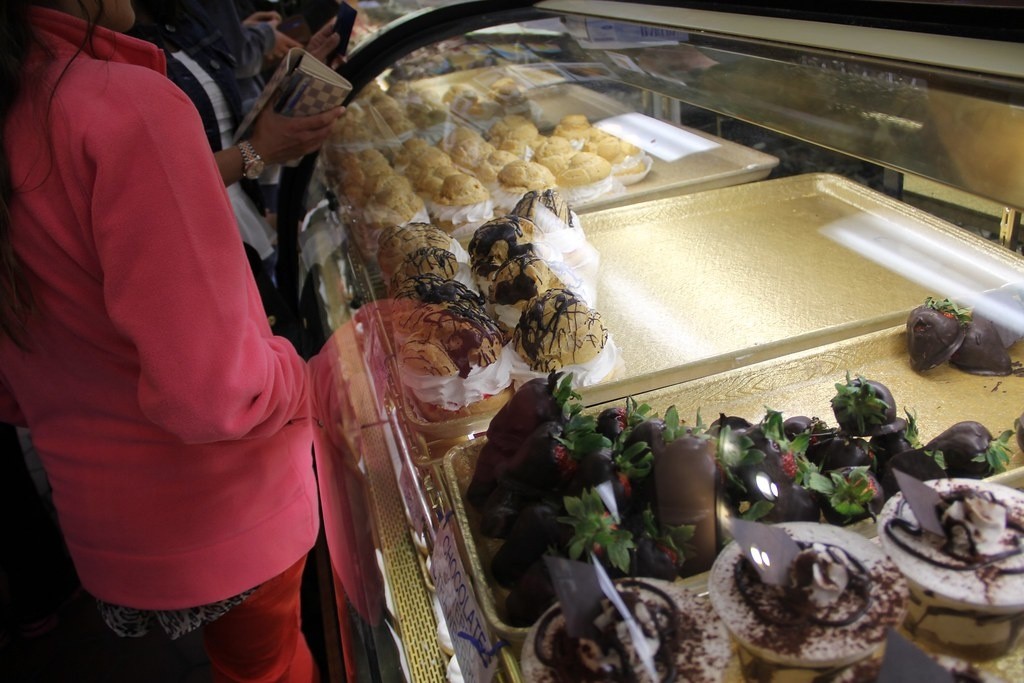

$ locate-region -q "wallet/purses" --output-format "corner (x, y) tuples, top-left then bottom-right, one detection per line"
(230, 46), (352, 167)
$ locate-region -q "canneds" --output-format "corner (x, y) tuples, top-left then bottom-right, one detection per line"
(326, 39), (655, 419)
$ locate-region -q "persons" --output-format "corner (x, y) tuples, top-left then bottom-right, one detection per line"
(0, 0), (320, 683)
(0, 423), (81, 638)
(639, 32), (885, 191)
(199, 0), (307, 195)
(120, 0), (348, 356)
(307, 299), (468, 629)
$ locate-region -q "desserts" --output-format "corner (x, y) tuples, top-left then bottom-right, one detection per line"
(464, 289), (1024, 683)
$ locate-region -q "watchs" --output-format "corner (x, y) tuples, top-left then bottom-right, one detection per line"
(237, 141), (265, 179)
